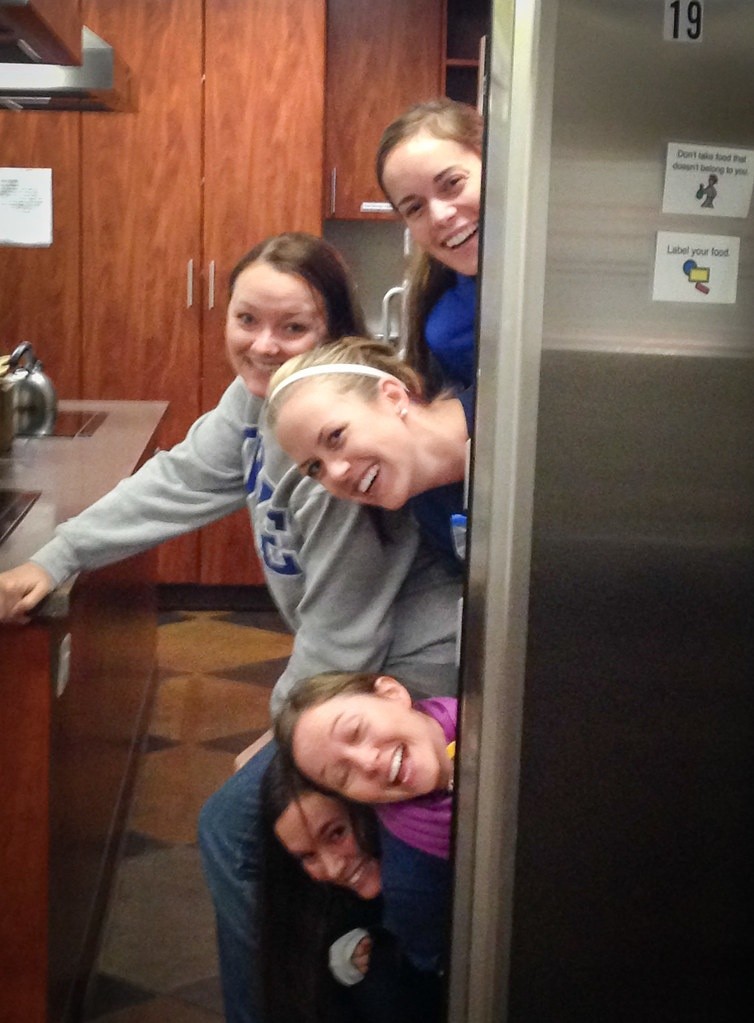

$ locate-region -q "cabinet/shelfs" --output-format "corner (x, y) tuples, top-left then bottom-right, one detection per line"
(1, 435), (161, 1023)
(1, 1), (487, 588)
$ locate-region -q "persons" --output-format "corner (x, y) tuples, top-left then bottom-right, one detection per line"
(376, 95), (482, 401)
(274, 674), (457, 981)
(259, 332), (476, 578)
(0, 233), (464, 1023)
(257, 749), (449, 1023)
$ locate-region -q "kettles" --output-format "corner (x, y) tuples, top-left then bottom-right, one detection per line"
(0, 341), (57, 436)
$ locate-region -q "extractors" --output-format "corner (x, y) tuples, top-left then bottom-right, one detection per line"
(0, 0), (138, 113)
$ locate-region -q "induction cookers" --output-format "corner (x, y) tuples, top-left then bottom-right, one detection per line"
(0, 490), (42, 544)
(20, 409), (108, 437)
